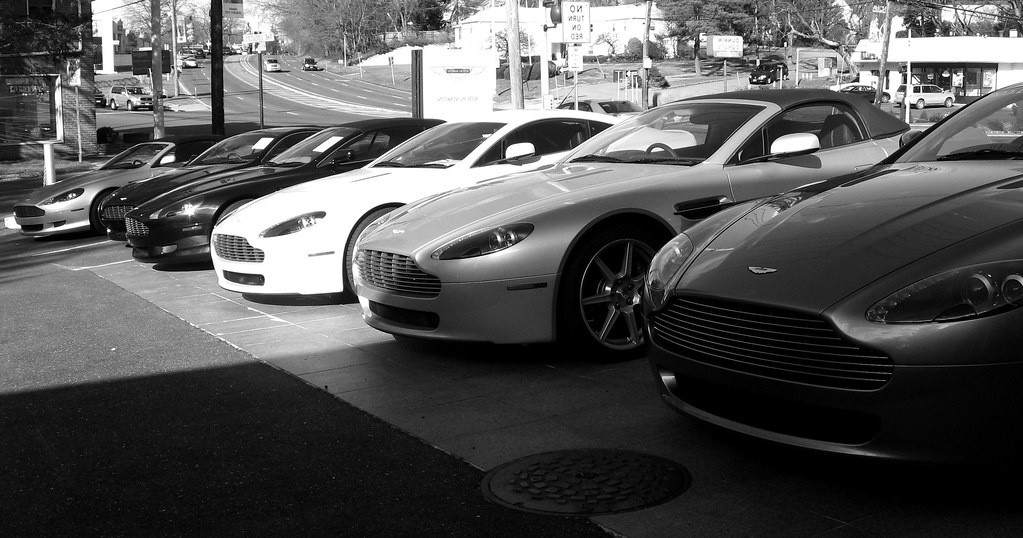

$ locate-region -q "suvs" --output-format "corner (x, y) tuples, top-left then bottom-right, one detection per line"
(106, 85), (153, 111)
(894, 84), (956, 109)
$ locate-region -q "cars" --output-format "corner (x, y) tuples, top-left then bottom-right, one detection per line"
(93, 87), (107, 109)
(222, 47), (242, 56)
(180, 45), (211, 69)
(264, 58), (282, 73)
(302, 57), (318, 71)
(748, 60), (789, 85)
(837, 85), (891, 103)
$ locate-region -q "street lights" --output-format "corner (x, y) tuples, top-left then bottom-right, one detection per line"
(795, 48), (799, 87)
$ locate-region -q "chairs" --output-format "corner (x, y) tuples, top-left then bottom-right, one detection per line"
(817, 112), (860, 144)
(550, 125), (584, 153)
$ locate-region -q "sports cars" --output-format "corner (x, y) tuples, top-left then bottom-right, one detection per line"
(98, 126), (330, 242)
(210, 108), (698, 301)
(637, 83), (1022, 528)
(350, 89), (991, 366)
(11, 134), (229, 238)
(123, 118), (445, 266)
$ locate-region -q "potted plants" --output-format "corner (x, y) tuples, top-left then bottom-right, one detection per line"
(97, 127), (114, 155)
